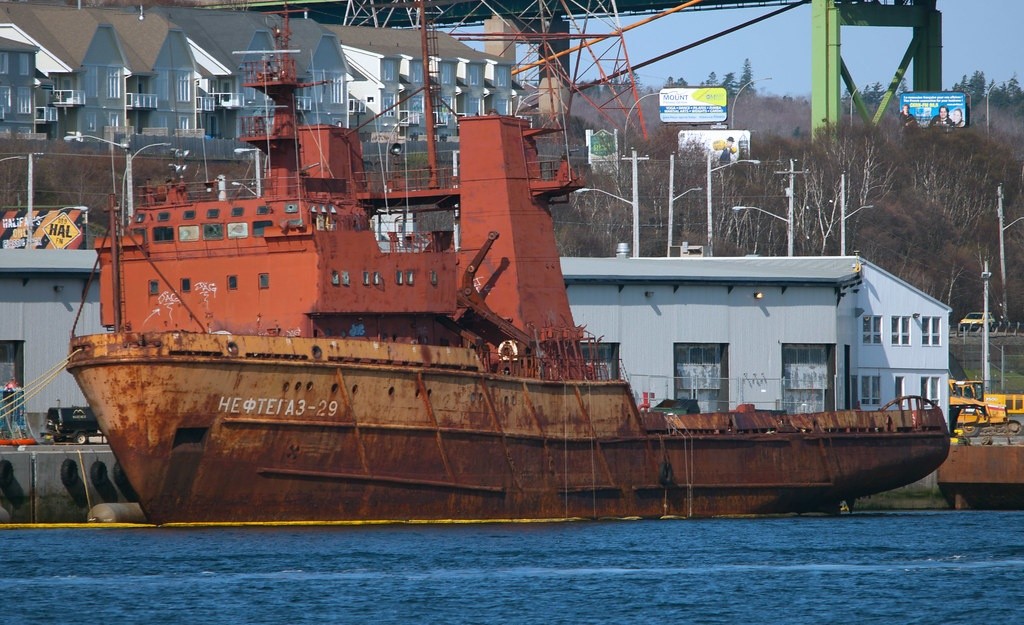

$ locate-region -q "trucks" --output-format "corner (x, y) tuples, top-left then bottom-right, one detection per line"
(44, 406), (100, 444)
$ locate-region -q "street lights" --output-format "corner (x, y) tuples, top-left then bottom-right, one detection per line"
(705, 158), (763, 254)
(664, 185), (702, 256)
(235, 146), (262, 200)
(731, 76), (773, 128)
(27, 206), (89, 250)
(986, 77), (1020, 146)
(120, 141), (173, 230)
(623, 92), (669, 157)
(231, 181), (257, 197)
(731, 204), (793, 256)
(386, 111), (424, 176)
(841, 205), (875, 254)
(574, 187), (640, 257)
(63, 134), (135, 225)
(849, 81), (879, 128)
(1000, 216), (1024, 321)
(981, 272), (992, 394)
(513, 88), (557, 117)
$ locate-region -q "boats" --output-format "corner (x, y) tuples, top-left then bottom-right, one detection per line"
(65, 0), (950, 525)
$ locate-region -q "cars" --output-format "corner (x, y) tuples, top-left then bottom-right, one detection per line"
(960, 311), (996, 333)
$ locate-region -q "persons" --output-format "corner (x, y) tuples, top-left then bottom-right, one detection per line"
(929, 106), (955, 127)
(900, 105), (917, 125)
(952, 109), (965, 127)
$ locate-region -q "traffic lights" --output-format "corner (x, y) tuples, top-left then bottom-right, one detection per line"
(391, 143), (402, 165)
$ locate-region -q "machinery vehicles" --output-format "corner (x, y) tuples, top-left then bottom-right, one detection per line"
(949, 379), (1024, 436)
